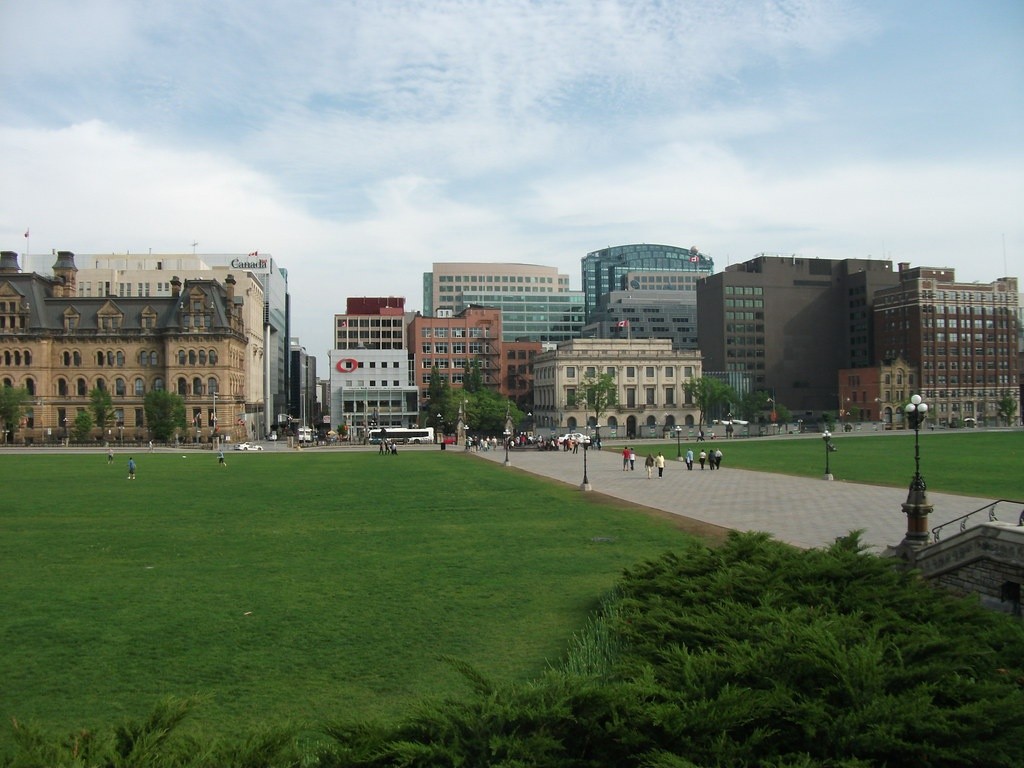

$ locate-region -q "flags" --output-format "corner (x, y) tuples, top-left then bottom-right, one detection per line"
(690, 257), (698, 262)
(24, 232), (29, 237)
(616, 320), (628, 327)
(248, 251), (257, 256)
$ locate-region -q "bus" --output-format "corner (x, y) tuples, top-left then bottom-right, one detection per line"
(368, 426), (434, 445)
(297, 426), (314, 443)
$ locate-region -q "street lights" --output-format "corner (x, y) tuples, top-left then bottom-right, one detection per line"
(118, 426), (125, 447)
(436, 413), (443, 433)
(577, 435), (591, 483)
(899, 394), (933, 540)
(300, 392), (306, 444)
(674, 425), (683, 457)
(464, 424), (470, 449)
(257, 397), (270, 442)
(767, 395), (776, 436)
(502, 428), (512, 461)
(213, 391), (219, 431)
(595, 423), (601, 445)
(662, 412), (671, 432)
(840, 394), (850, 432)
(727, 413), (732, 431)
(526, 412), (533, 431)
(62, 417), (69, 437)
(211, 416), (221, 437)
(822, 430), (833, 474)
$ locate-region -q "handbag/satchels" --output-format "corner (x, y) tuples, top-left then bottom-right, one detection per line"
(686, 456), (690, 463)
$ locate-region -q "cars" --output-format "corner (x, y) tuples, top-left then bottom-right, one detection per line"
(233, 441), (264, 451)
(558, 433), (583, 444)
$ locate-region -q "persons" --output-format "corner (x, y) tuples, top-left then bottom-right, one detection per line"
(126, 457), (136, 480)
(708, 448), (723, 470)
(645, 453), (655, 479)
(378, 441), (397, 455)
(685, 448), (693, 470)
(107, 448), (114, 464)
(931, 424), (934, 431)
(696, 430), (705, 442)
(699, 449), (706, 470)
(654, 452), (665, 478)
(562, 437), (579, 454)
(466, 435), (497, 452)
(622, 447), (630, 471)
(629, 447), (636, 470)
(148, 439), (153, 452)
(508, 434), (560, 451)
(217, 450), (226, 467)
